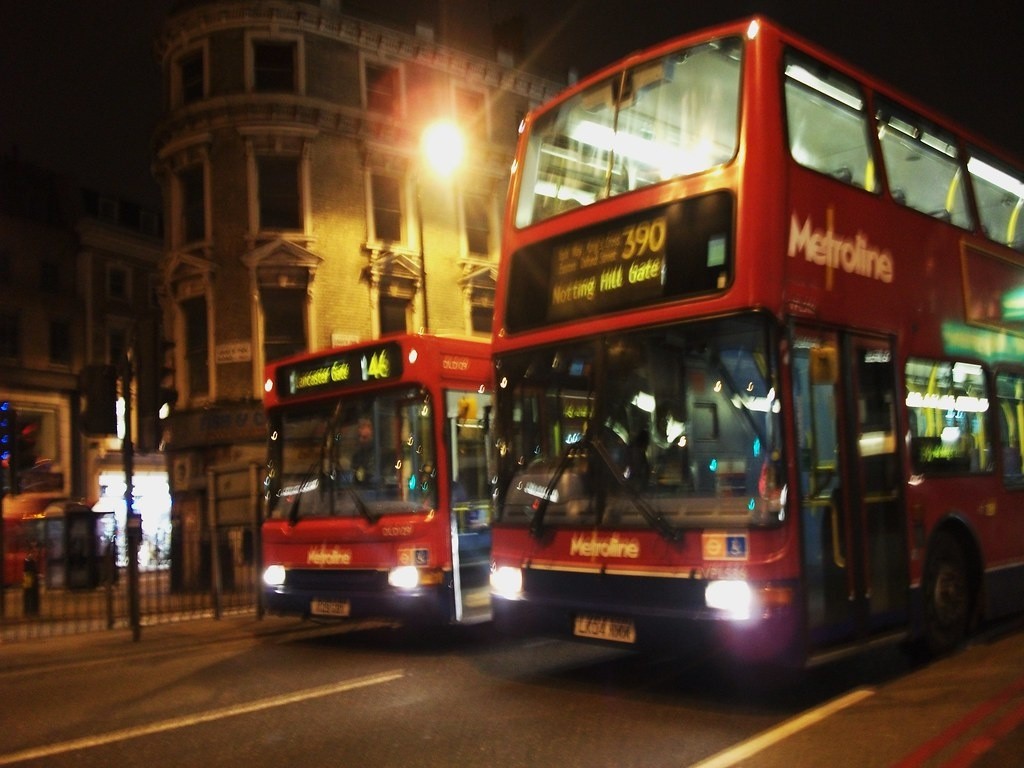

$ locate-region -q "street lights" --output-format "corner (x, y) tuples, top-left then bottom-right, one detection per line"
(410, 115), (466, 329)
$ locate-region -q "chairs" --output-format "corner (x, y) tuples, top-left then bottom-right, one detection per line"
(825, 166), (1024, 251)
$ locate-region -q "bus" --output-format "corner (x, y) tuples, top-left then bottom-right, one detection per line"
(0, 500), (119, 592)
(487, 14), (1024, 676)
(254, 334), (603, 637)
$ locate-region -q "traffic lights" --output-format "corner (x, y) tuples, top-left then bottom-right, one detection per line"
(129, 305), (179, 455)
(10, 413), (41, 470)
